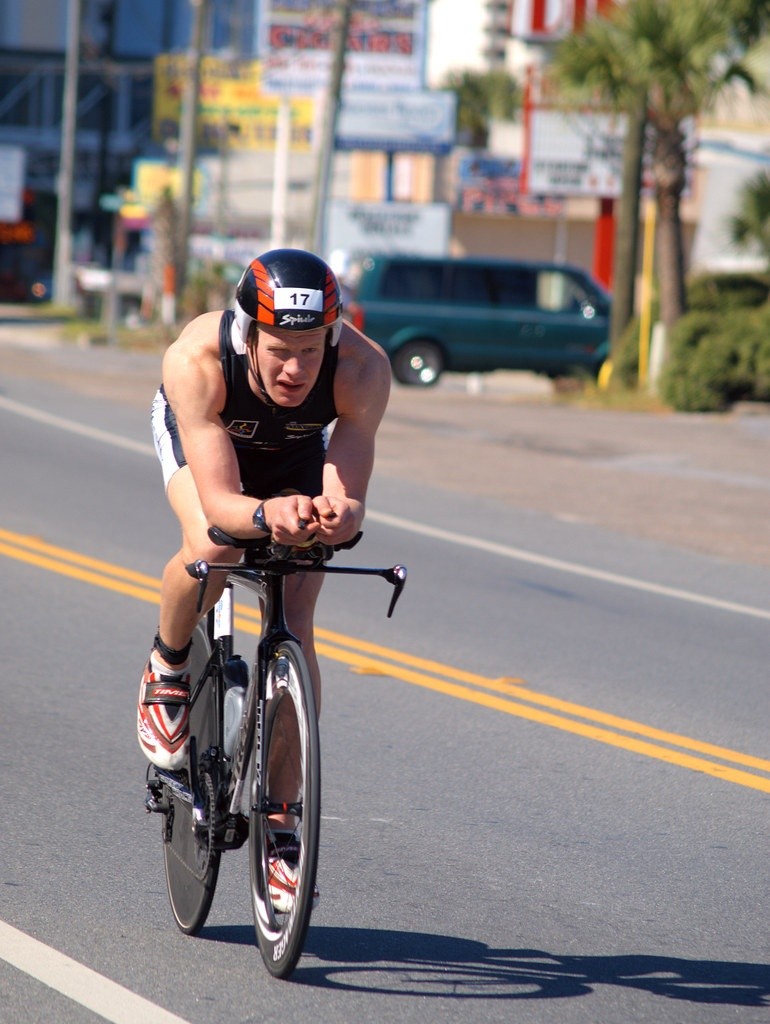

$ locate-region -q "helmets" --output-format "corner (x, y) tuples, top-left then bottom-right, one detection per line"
(231, 248), (345, 355)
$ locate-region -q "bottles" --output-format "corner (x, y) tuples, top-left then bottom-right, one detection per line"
(225, 656), (249, 693)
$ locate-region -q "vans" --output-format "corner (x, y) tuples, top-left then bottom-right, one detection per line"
(329, 247), (616, 394)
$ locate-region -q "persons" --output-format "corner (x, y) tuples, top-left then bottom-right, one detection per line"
(134, 249), (392, 915)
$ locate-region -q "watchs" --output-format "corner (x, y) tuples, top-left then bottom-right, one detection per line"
(252, 498), (272, 534)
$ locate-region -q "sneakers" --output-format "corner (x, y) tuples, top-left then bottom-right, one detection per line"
(135, 658), (192, 771)
(265, 832), (320, 914)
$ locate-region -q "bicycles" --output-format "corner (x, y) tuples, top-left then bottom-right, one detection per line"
(143, 520), (409, 978)
